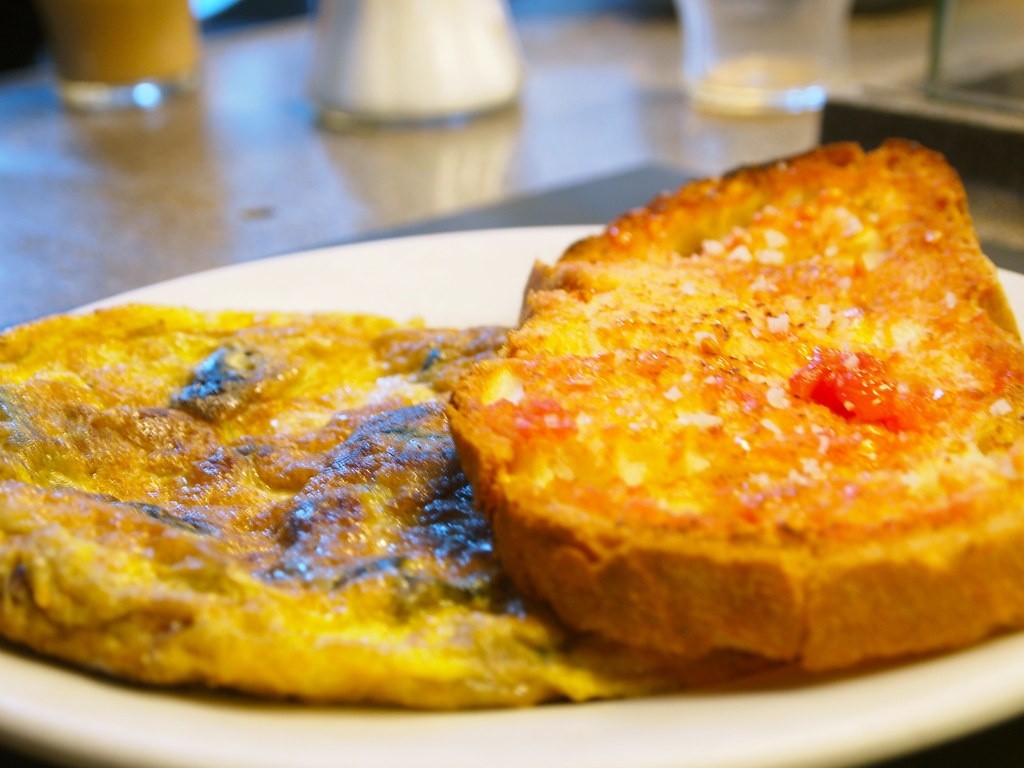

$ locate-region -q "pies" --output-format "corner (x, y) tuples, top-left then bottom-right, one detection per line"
(2, 303), (624, 708)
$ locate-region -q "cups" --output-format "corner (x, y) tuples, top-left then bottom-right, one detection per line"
(46, 0), (207, 106)
(313, 0), (521, 134)
(674, 0), (853, 118)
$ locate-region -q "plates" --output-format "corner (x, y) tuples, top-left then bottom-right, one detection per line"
(0, 225), (1024, 768)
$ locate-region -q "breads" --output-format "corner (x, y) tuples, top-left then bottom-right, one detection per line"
(447, 137), (1024, 669)
